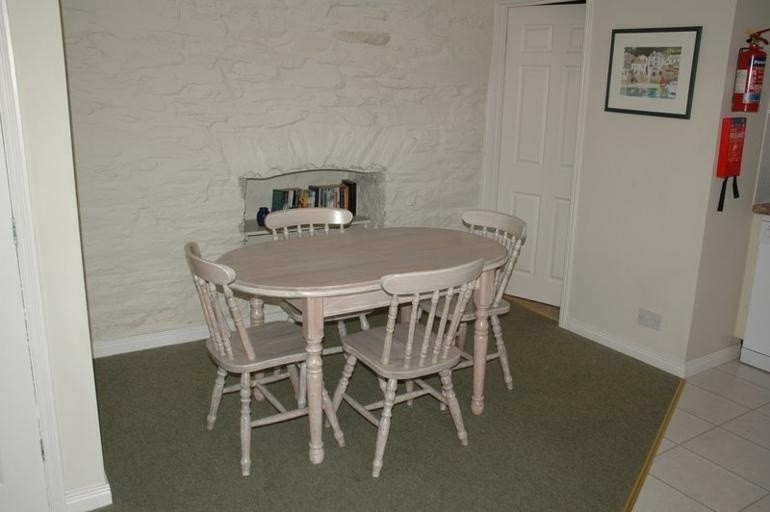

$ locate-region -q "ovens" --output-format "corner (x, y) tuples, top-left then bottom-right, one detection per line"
(740, 220), (770, 372)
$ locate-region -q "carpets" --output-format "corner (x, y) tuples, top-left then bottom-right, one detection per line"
(76, 287), (686, 510)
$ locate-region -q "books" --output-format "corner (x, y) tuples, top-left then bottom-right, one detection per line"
(270, 178), (357, 220)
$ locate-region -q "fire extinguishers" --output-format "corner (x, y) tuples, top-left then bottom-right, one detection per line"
(732, 28), (770, 112)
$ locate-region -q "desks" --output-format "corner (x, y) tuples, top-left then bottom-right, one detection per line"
(210, 227), (508, 465)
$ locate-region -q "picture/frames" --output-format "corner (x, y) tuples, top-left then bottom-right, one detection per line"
(602, 25), (704, 120)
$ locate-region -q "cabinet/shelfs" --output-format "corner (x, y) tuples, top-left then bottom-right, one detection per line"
(738, 202), (770, 373)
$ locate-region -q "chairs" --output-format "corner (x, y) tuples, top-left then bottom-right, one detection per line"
(264, 205), (392, 409)
(324, 257), (490, 478)
(419, 207), (532, 410)
(183, 238), (348, 478)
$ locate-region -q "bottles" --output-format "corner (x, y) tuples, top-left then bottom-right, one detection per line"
(257, 207), (270, 227)
